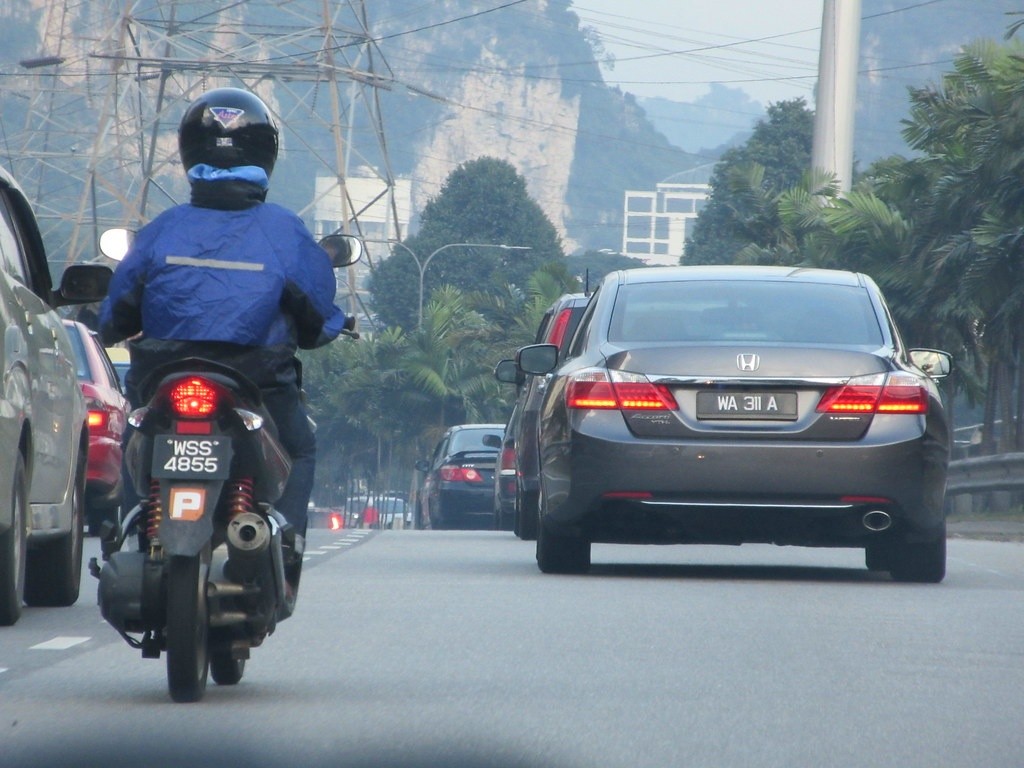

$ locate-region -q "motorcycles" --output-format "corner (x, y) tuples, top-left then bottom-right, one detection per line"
(88, 228), (363, 705)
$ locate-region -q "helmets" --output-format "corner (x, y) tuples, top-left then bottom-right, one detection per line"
(322, 237), (353, 265)
(178, 88), (278, 180)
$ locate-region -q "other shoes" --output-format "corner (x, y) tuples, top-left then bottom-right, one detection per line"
(282, 544), (299, 588)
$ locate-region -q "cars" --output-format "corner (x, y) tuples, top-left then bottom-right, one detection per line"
(308, 482), (411, 535)
(413, 263), (954, 582)
(0, 168), (129, 626)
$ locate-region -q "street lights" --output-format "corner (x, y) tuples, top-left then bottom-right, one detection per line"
(351, 239), (532, 333)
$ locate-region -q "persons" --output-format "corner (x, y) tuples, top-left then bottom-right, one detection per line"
(96, 87), (345, 608)
